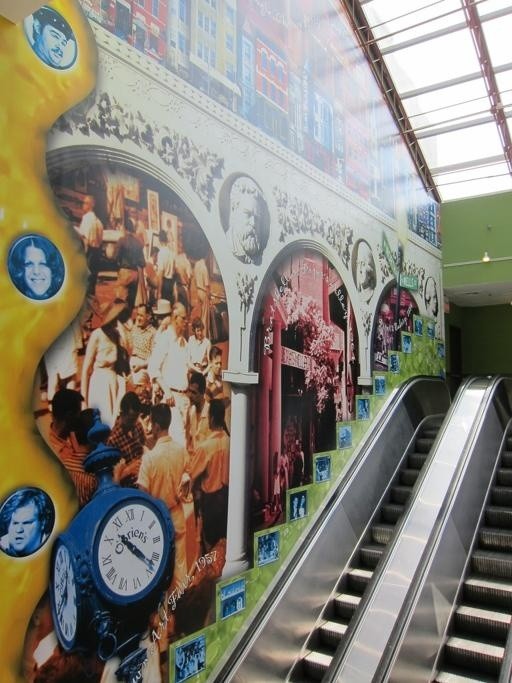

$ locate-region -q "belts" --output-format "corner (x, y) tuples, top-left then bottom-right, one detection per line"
(171, 389), (188, 393)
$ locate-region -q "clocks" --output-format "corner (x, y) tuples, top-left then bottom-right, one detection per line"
(47, 405), (181, 683)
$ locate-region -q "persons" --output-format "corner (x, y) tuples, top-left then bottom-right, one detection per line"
(30, 5), (75, 64)
(425, 277), (437, 316)
(290, 496), (299, 519)
(10, 236), (60, 299)
(355, 241), (375, 303)
(2, 489), (48, 552)
(226, 177), (268, 264)
(298, 495), (306, 517)
(173, 639), (207, 682)
(272, 438), (306, 512)
(33, 195), (230, 644)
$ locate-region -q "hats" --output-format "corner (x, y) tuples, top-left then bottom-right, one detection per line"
(153, 299), (172, 313)
(90, 302), (124, 329)
